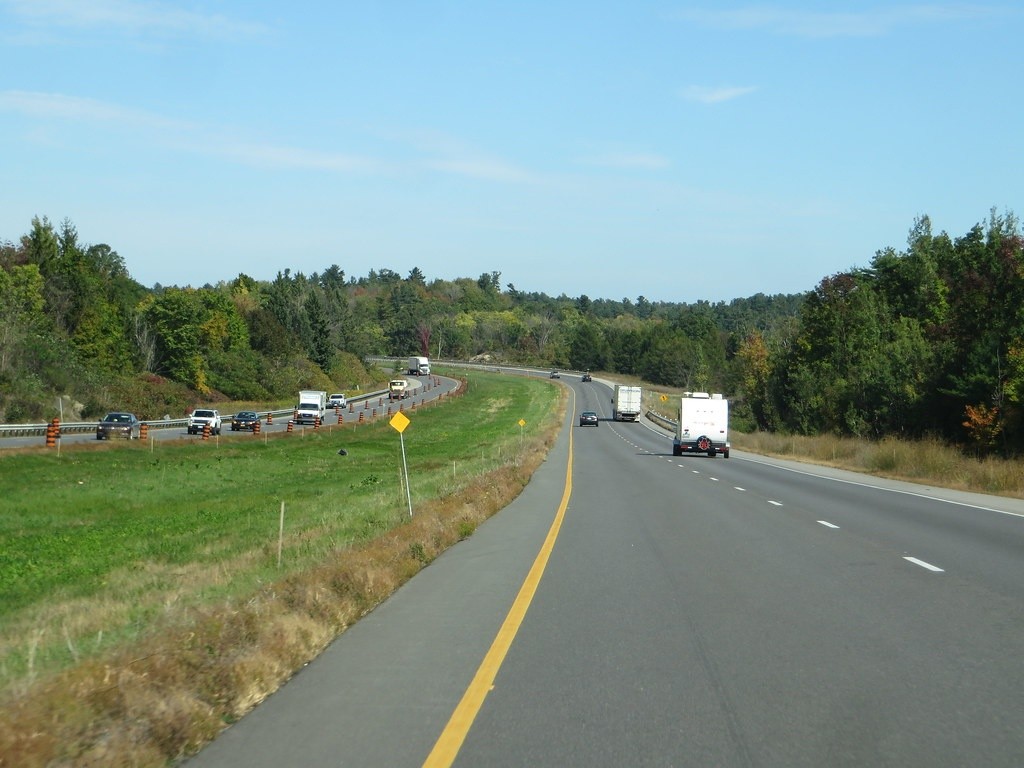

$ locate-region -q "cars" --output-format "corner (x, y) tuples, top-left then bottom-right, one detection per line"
(579, 411), (599, 427)
(232, 411), (261, 431)
(582, 374), (591, 382)
(97, 412), (142, 440)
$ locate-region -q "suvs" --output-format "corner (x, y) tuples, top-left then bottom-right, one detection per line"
(188, 408), (222, 435)
(550, 370), (560, 379)
(326, 394), (347, 409)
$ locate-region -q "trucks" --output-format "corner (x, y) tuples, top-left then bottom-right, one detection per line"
(292, 390), (325, 425)
(388, 379), (409, 399)
(673, 391), (730, 459)
(408, 356), (431, 376)
(610, 384), (642, 423)
